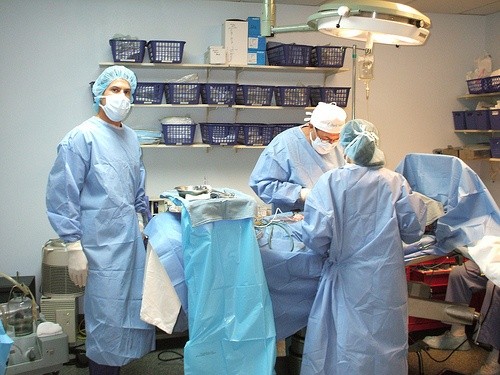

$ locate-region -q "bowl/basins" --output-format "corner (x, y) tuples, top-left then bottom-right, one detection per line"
(175, 186), (208, 197)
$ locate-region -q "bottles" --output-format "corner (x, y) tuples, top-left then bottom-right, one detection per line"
(7, 287), (34, 337)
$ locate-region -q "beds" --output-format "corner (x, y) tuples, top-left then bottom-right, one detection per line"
(140, 153), (500, 342)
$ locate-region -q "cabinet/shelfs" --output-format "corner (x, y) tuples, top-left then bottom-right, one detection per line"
(453, 92), (500, 162)
(99, 62), (349, 149)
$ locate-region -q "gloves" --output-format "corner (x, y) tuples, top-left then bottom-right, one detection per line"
(66, 240), (89, 288)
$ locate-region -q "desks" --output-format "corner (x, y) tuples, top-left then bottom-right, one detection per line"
(160, 188), (258, 227)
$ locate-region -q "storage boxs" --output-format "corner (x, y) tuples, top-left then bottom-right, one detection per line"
(205, 16), (346, 67)
(133, 82), (351, 108)
(108, 39), (186, 63)
(160, 123), (303, 145)
(443, 75), (500, 159)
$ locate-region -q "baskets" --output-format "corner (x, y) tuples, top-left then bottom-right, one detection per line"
(465, 75), (499, 94)
(202, 82), (240, 106)
(158, 122), (198, 145)
(237, 84), (275, 106)
(312, 45), (347, 68)
(235, 123), (274, 146)
(269, 122), (304, 140)
(199, 121), (241, 146)
(164, 82), (202, 105)
(146, 39), (187, 64)
(130, 81), (165, 105)
(265, 41), (314, 67)
(109, 38), (148, 63)
(308, 86), (352, 108)
(274, 85), (311, 107)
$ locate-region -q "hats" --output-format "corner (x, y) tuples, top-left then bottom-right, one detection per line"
(311, 101), (347, 135)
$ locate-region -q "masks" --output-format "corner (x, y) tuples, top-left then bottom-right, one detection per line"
(310, 127), (340, 155)
(97, 96), (132, 123)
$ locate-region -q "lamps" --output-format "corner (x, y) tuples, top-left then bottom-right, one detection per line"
(260, 1), (431, 53)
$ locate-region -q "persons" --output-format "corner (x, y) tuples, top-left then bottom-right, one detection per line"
(45, 66), (157, 375)
(248, 101), (348, 214)
(298, 118), (428, 375)
(423, 259), (500, 375)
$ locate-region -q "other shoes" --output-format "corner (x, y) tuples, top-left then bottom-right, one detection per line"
(473, 363), (500, 375)
(422, 330), (470, 351)
